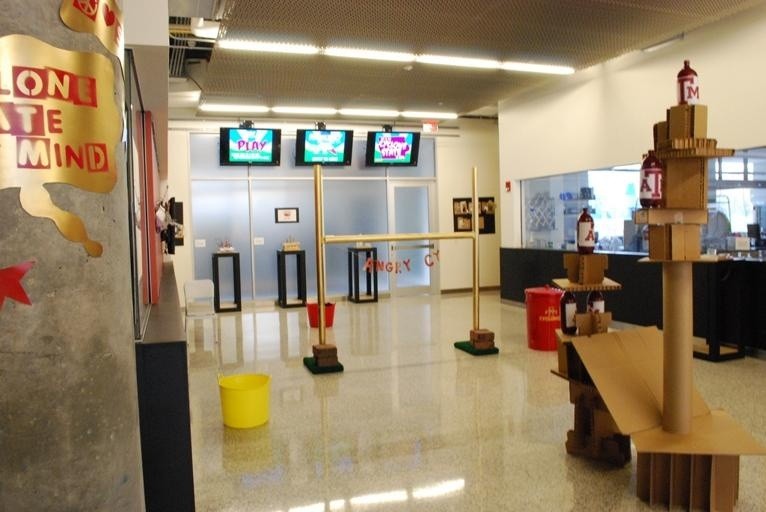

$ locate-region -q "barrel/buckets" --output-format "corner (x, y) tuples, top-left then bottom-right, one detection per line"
(216, 370), (273, 428)
(305, 302), (334, 327)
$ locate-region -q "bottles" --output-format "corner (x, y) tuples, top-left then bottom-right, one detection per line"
(576, 207), (595, 254)
(587, 291), (605, 313)
(560, 291), (578, 335)
(640, 151), (664, 208)
(677, 60), (699, 105)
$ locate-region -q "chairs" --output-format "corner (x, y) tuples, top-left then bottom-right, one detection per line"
(182, 278), (219, 344)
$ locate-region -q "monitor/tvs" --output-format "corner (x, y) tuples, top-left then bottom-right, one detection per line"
(295, 129), (353, 166)
(220, 127), (281, 165)
(366, 131), (420, 166)
